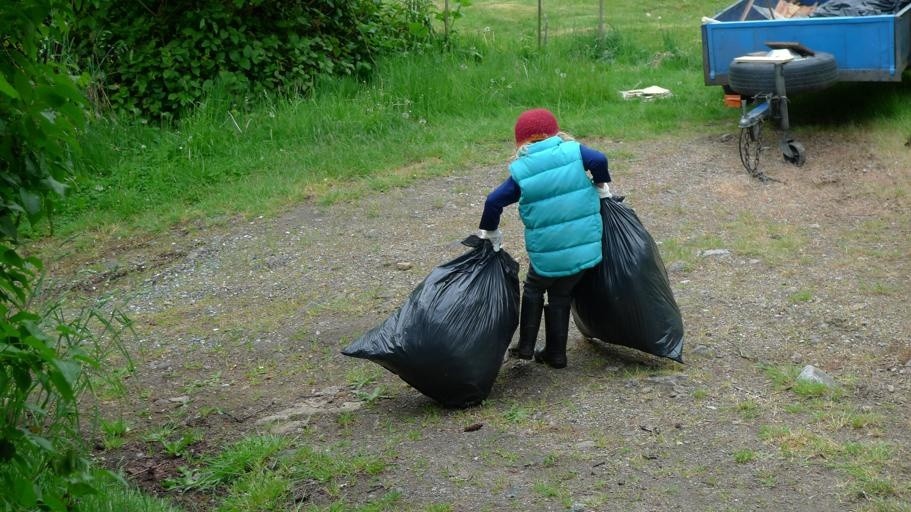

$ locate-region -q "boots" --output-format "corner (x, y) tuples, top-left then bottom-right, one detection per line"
(508, 297), (542, 359)
(536, 304), (571, 370)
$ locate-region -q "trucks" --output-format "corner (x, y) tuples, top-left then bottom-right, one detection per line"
(700, 0), (911, 107)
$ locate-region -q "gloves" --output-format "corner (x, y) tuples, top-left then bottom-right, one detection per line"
(479, 229), (501, 254)
(594, 183), (611, 202)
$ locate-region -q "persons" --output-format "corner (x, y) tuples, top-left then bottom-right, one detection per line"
(479, 108), (614, 369)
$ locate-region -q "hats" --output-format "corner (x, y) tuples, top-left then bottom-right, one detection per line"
(516, 110), (558, 148)
